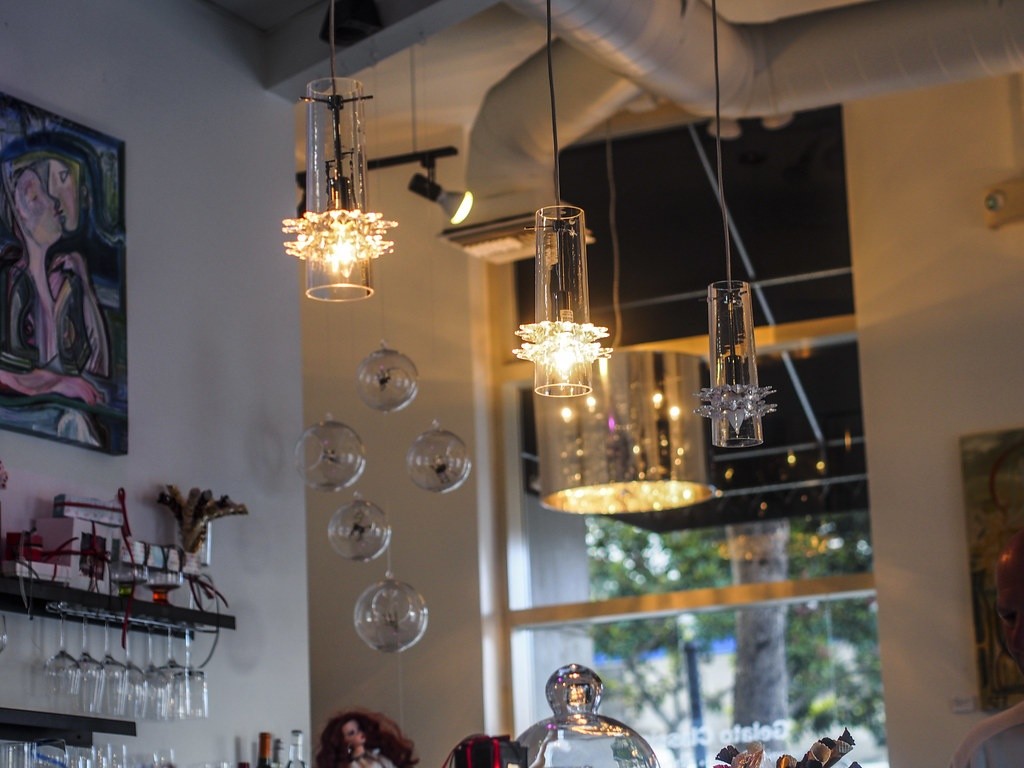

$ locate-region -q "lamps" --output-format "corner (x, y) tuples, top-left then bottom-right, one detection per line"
(511, 0), (613, 399)
(280, 0), (399, 302)
(532, 350), (707, 516)
(407, 158), (474, 224)
(691, 1), (779, 449)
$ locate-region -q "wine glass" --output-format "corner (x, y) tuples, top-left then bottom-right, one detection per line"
(43, 601), (208, 721)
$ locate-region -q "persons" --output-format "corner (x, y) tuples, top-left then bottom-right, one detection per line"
(316, 712), (419, 768)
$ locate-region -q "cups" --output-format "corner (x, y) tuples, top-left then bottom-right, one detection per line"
(0, 736), (231, 768)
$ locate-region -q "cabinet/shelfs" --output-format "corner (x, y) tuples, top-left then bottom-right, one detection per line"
(0, 573), (236, 748)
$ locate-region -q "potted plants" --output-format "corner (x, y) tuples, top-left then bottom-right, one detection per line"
(156, 482), (248, 573)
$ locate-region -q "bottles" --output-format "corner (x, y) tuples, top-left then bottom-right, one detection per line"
(237, 729), (307, 768)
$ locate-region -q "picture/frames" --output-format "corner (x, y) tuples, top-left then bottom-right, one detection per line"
(0, 91), (130, 457)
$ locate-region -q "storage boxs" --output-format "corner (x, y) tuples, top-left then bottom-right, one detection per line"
(0, 559), (71, 582)
(36, 517), (112, 595)
